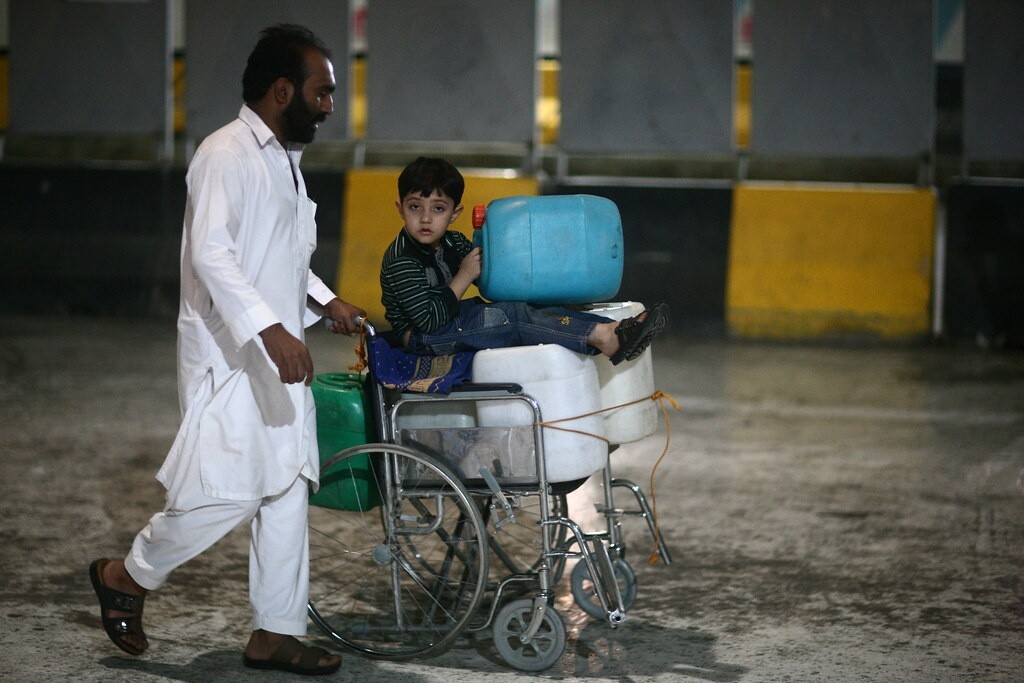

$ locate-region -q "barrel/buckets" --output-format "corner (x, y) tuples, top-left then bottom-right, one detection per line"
(309, 373), (387, 512)
(579, 302), (660, 445)
(390, 389), (479, 484)
(472, 342), (610, 483)
(473, 194), (624, 305)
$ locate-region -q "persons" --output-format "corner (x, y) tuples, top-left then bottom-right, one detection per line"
(88, 21), (367, 677)
(379, 158), (675, 367)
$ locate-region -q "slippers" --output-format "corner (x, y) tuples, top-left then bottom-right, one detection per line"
(609, 300), (669, 364)
(243, 635), (342, 674)
(89, 557), (149, 655)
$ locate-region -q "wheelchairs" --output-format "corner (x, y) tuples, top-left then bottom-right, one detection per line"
(302, 316), (672, 673)
